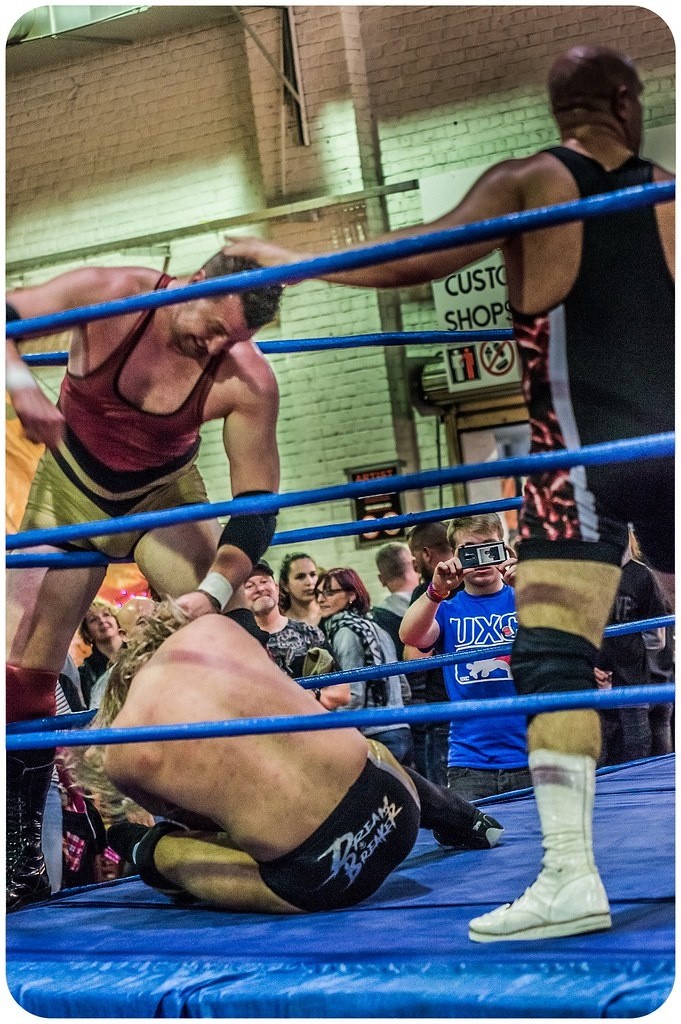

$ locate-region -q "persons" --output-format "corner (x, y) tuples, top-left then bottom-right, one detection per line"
(38, 591), (184, 897)
(242, 556), (352, 714)
(273, 551), (330, 636)
(4, 248), (284, 919)
(220, 38), (675, 942)
(592, 527), (676, 769)
(400, 520), (465, 792)
(371, 537), (430, 786)
(312, 565), (420, 778)
(83, 597), (507, 916)
(219, 574), (273, 650)
(398, 512), (534, 807)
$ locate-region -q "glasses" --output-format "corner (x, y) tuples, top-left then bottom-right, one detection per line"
(314, 587), (345, 598)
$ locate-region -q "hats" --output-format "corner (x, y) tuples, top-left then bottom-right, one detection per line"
(254, 558), (275, 575)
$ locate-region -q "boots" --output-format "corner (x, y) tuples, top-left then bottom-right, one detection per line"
(467, 749), (613, 946)
(6, 755), (58, 913)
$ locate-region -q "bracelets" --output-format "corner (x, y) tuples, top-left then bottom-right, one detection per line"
(4, 363), (33, 400)
(425, 582), (451, 603)
(194, 571), (231, 613)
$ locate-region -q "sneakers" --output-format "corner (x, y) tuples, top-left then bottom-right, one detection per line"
(434, 808), (505, 850)
(104, 820), (151, 866)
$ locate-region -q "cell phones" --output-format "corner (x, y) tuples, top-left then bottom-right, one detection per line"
(457, 539), (507, 570)
